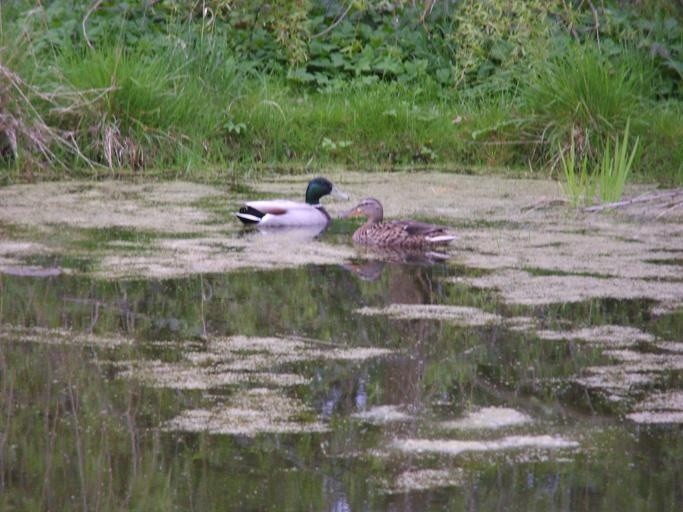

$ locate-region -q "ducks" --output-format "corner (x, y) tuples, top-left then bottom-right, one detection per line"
(347, 198), (461, 250)
(232, 177), (348, 227)
